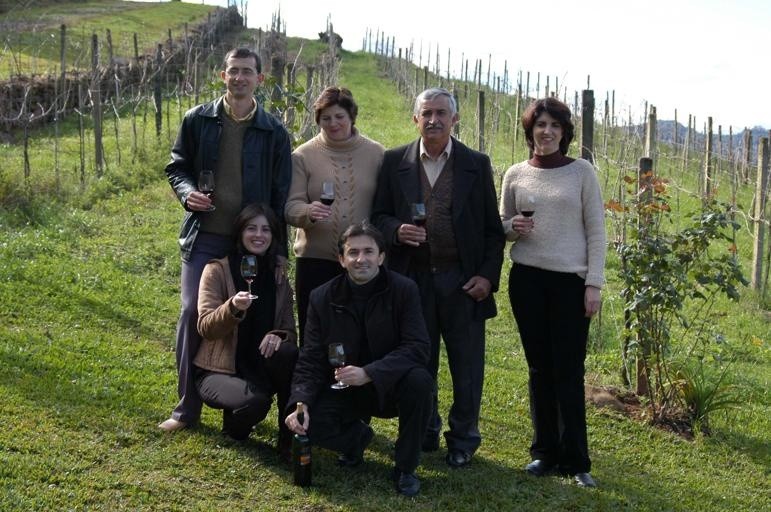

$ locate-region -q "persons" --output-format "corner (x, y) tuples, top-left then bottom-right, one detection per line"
(371, 88), (507, 466)
(280, 220), (433, 494)
(158, 47), (292, 433)
(283, 85), (385, 346)
(499, 98), (604, 490)
(191, 204), (300, 465)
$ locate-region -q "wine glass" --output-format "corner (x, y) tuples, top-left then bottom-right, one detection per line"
(328, 342), (350, 390)
(411, 203), (428, 243)
(319, 181), (336, 206)
(197, 170), (216, 211)
(520, 195), (536, 232)
(240, 255), (259, 300)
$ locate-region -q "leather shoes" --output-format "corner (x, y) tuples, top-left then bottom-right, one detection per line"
(342, 426), (374, 467)
(421, 431), (439, 453)
(394, 464), (421, 496)
(445, 449), (472, 467)
(574, 471), (595, 488)
(524, 459), (548, 478)
(158, 418), (188, 433)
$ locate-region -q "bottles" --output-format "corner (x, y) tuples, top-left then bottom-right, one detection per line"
(293, 402), (311, 487)
